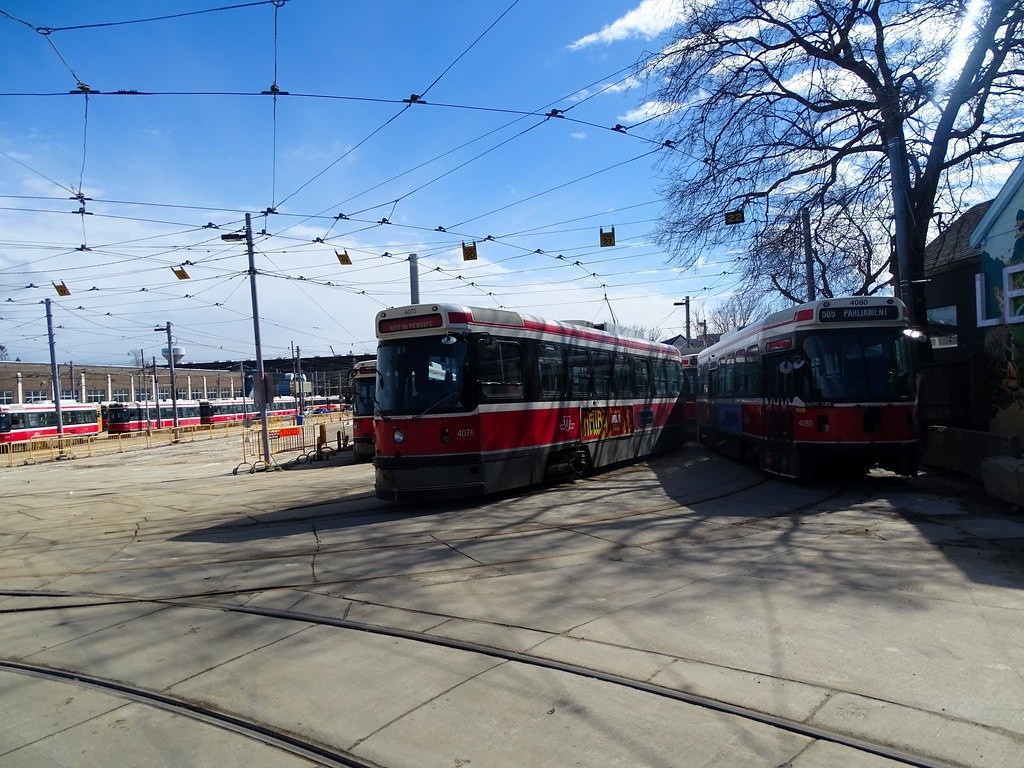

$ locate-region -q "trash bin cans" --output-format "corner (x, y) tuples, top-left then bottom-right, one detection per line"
(297, 415), (304, 425)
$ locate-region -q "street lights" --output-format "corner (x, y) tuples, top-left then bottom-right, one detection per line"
(154, 321), (180, 442)
(673, 296), (691, 349)
(221, 213), (272, 469)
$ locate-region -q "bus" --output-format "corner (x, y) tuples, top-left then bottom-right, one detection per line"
(0, 399), (102, 444)
(697, 296), (928, 484)
(343, 361), (457, 465)
(680, 354), (699, 441)
(101, 395), (354, 435)
(374, 304), (687, 502)
